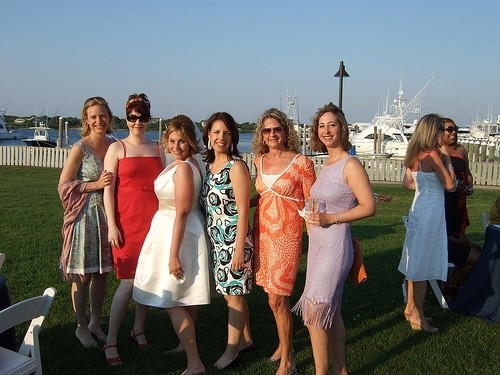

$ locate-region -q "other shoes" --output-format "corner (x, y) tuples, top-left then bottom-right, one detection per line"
(238, 339), (252, 351)
(269, 347), (293, 362)
(275, 363), (297, 375)
(214, 350), (238, 370)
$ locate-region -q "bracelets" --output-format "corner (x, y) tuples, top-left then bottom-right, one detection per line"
(334, 213), (339, 224)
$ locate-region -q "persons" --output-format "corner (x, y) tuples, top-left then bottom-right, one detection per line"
(104, 92), (165, 370)
(132, 114), (210, 375)
(202, 111), (254, 375)
(292, 104), (375, 375)
(58, 97), (117, 352)
(398, 113), (482, 332)
(249, 107), (316, 375)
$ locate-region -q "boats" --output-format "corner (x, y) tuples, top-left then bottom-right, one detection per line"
(277, 72), (500, 172)
(0, 117), (22, 140)
(20, 106), (56, 147)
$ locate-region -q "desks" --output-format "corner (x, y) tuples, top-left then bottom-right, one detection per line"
(448, 224), (500, 323)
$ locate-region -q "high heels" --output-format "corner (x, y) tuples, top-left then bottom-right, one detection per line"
(129, 329), (149, 351)
(102, 341), (122, 367)
(404, 313), (432, 322)
(409, 321), (438, 333)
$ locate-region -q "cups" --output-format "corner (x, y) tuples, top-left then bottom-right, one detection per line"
(305, 197), (319, 214)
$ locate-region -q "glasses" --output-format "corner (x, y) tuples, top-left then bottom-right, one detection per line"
(127, 114), (149, 123)
(262, 126), (282, 135)
(445, 125), (458, 133)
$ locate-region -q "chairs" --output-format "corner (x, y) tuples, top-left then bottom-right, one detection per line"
(0, 287), (57, 375)
(402, 216), (456, 309)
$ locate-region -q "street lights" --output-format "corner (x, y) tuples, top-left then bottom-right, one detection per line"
(334, 60), (350, 110)
